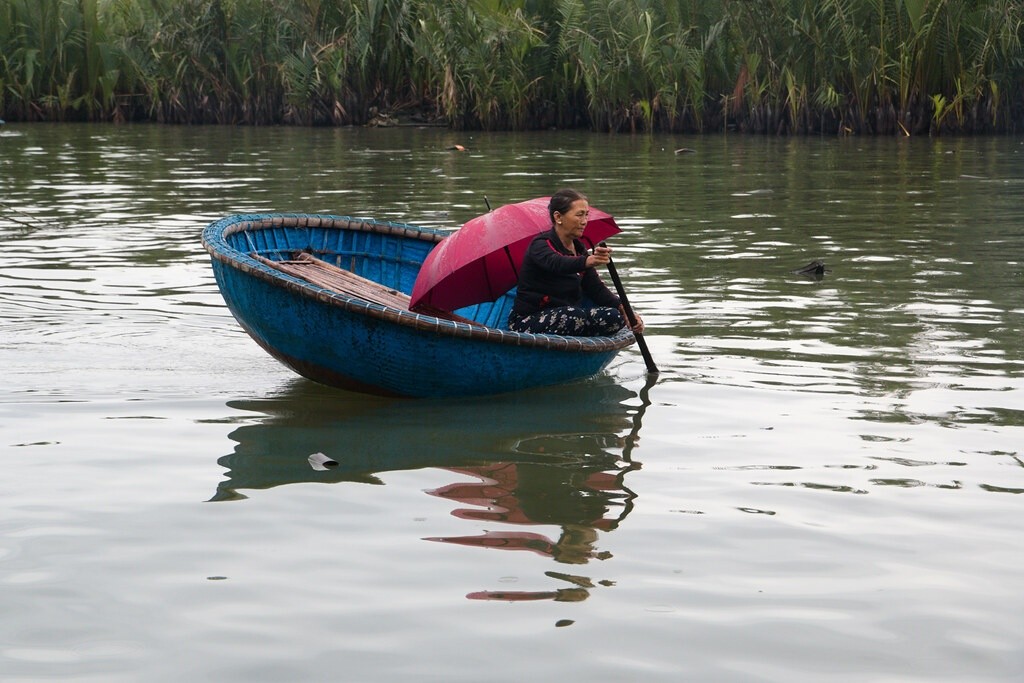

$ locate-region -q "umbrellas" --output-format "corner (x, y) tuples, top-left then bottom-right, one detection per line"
(408, 195), (623, 320)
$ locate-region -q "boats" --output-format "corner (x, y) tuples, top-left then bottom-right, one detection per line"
(200, 209), (645, 403)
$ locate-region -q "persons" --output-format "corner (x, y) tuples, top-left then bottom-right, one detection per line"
(505, 186), (645, 338)
